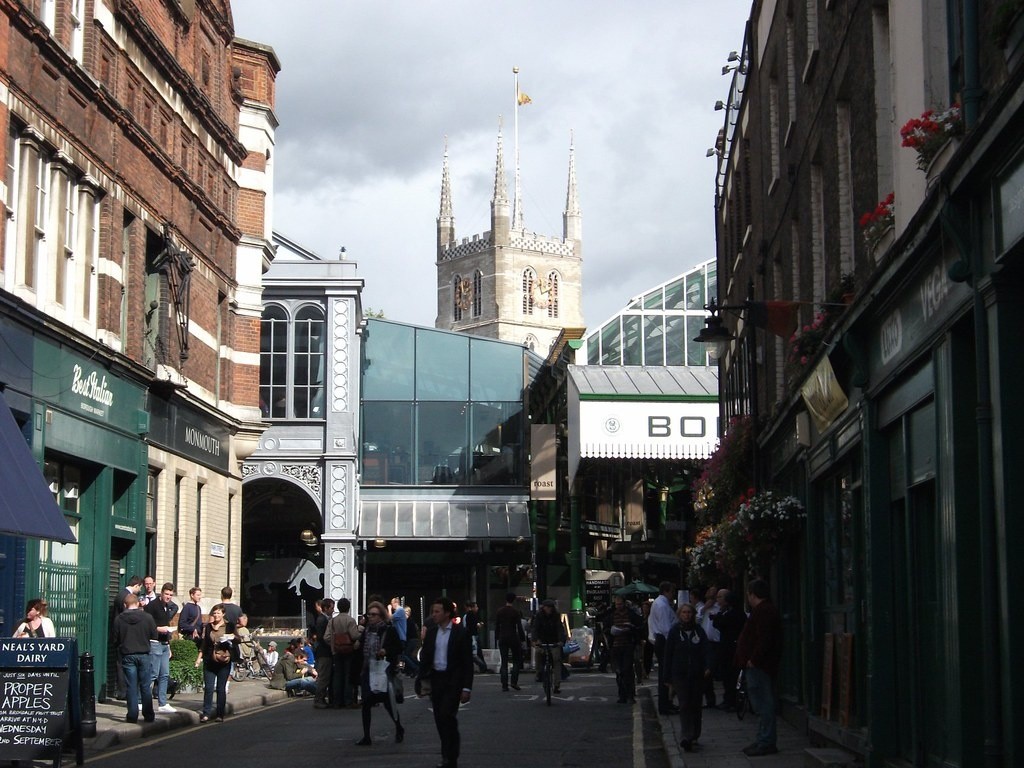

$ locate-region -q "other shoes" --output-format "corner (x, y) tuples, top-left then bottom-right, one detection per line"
(126, 713), (137, 723)
(215, 717), (223, 722)
(724, 706), (743, 712)
(554, 689), (561, 694)
(743, 743), (778, 756)
(144, 713), (154, 721)
(356, 737), (371, 745)
(660, 708), (681, 715)
(201, 714), (210, 722)
(628, 698), (636, 704)
(680, 739), (703, 749)
(313, 698), (329, 708)
(502, 684), (509, 691)
(617, 698), (627, 703)
(685, 743), (698, 753)
(511, 683), (521, 690)
(702, 704), (715, 707)
(659, 704), (680, 710)
(716, 702), (731, 709)
(396, 727), (404, 743)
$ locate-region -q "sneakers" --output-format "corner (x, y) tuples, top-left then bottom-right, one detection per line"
(158, 704), (177, 712)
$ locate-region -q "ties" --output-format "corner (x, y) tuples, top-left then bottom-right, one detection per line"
(147, 597), (153, 602)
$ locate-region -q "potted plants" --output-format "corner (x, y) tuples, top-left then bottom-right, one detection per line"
(828, 272), (856, 305)
(339, 247), (347, 261)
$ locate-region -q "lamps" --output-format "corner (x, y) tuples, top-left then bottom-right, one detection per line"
(515, 529), (524, 535)
(300, 529), (317, 546)
(375, 540), (386, 547)
(706, 52), (748, 160)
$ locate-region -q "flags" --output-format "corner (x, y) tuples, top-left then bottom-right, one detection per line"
(517, 74), (533, 107)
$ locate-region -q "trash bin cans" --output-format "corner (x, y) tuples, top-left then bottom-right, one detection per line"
(568, 626), (594, 667)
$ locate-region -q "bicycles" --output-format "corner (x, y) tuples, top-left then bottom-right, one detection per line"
(733, 640), (750, 720)
(593, 622), (610, 662)
(532, 643), (566, 706)
(228, 625), (273, 682)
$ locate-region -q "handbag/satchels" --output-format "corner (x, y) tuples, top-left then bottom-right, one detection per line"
(213, 648), (230, 663)
(369, 655), (389, 693)
(563, 638), (579, 653)
(334, 633), (353, 651)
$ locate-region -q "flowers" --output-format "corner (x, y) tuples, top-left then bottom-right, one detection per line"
(899, 99), (964, 172)
(787, 308), (832, 369)
(685, 413), (807, 590)
(860, 191), (895, 263)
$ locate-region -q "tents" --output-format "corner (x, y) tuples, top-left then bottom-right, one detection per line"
(613, 580), (661, 594)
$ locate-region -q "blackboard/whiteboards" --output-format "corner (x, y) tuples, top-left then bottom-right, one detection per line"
(0, 666), (83, 760)
(586, 580), (611, 608)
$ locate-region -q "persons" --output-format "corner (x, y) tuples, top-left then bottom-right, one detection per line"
(270, 649), (318, 696)
(208, 586), (243, 696)
(732, 579), (782, 756)
(195, 605), (234, 722)
(178, 587), (201, 651)
(324, 597), (360, 710)
(663, 604), (712, 753)
(420, 597), (474, 768)
(528, 600), (568, 705)
(113, 595), (159, 721)
(494, 593), (529, 692)
(313, 599), (334, 708)
(648, 581), (680, 714)
(12, 599), (56, 638)
(100, 495), (748, 768)
(356, 601), (407, 747)
(145, 583), (179, 712)
(603, 597), (645, 705)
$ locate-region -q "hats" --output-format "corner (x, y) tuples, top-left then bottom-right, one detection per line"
(268, 641), (278, 647)
(543, 600), (554, 606)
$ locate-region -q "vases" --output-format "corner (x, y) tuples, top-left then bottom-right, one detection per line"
(872, 225), (895, 265)
(923, 135), (964, 189)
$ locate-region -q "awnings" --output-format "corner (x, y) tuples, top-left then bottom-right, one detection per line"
(0, 390), (80, 543)
